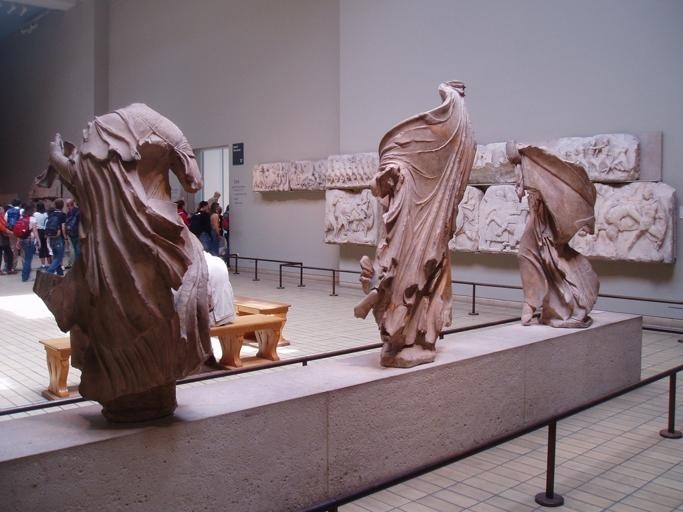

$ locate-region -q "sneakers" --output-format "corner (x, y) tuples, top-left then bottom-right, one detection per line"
(40, 263), (71, 277)
(0, 270), (17, 275)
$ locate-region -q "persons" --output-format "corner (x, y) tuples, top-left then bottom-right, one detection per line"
(0, 193), (229, 281)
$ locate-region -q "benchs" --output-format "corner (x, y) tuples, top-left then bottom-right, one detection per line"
(233, 295), (291, 348)
(39, 314), (288, 402)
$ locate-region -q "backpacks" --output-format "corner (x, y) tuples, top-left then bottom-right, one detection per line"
(46, 214), (61, 238)
(0, 208), (32, 245)
(66, 209), (79, 238)
